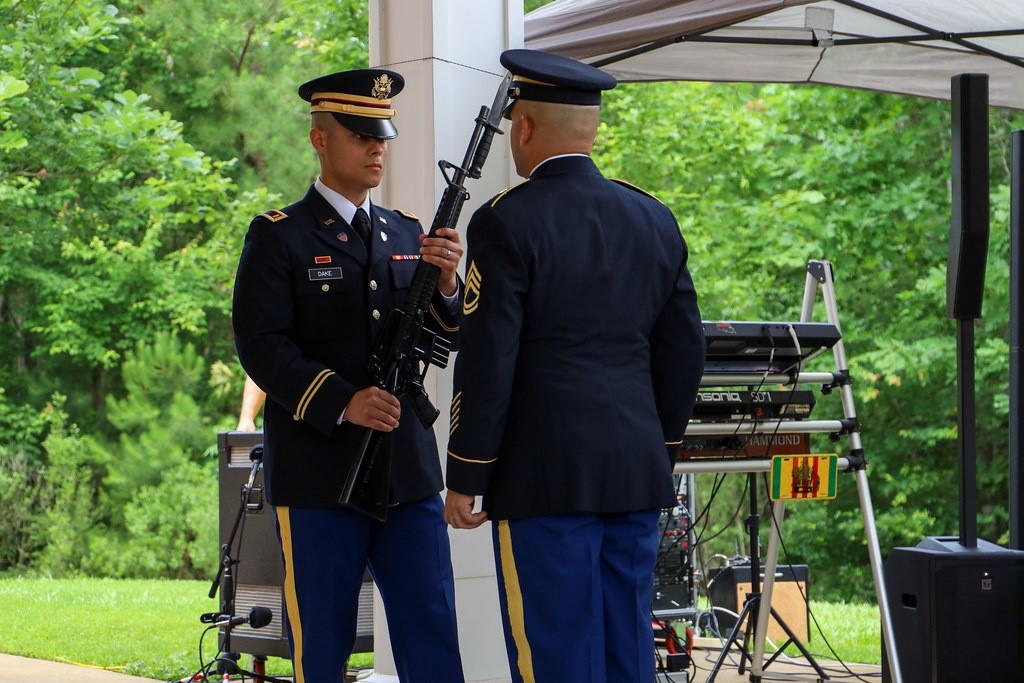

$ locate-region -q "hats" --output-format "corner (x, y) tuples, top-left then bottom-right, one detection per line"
(496, 48), (618, 118)
(296, 67), (405, 141)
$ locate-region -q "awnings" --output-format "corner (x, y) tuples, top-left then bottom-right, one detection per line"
(524, 0), (1024, 110)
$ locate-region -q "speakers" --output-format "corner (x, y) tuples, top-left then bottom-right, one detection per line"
(882, 536), (1024, 682)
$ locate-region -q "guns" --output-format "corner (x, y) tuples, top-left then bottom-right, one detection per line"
(331, 70), (514, 502)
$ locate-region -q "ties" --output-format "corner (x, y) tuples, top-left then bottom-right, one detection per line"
(346, 207), (375, 262)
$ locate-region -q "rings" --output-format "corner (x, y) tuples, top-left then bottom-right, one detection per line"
(446, 250), (450, 259)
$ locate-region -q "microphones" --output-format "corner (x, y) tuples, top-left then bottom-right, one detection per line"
(215, 606), (272, 628)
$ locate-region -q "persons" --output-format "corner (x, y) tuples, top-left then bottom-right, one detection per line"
(446, 49), (705, 683)
(236, 375), (266, 433)
(231, 69), (464, 683)
